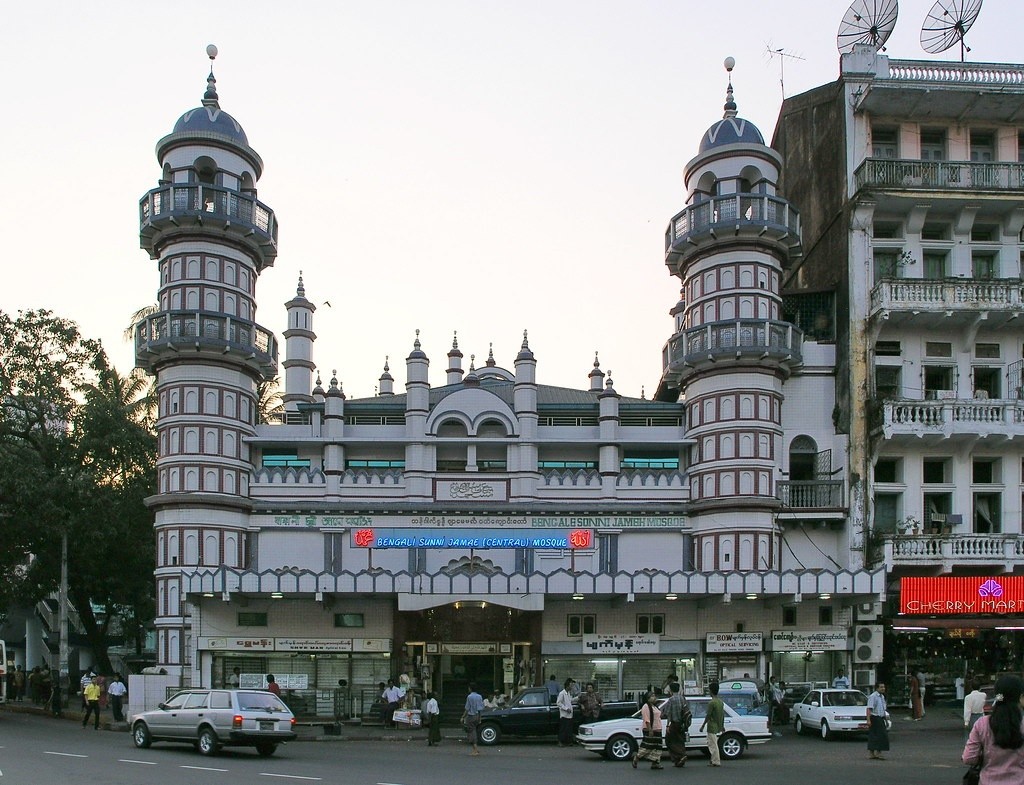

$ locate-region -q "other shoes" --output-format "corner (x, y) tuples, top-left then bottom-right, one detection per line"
(876, 755), (887, 760)
(914, 718), (922, 721)
(869, 755), (877, 759)
(429, 742), (434, 746)
(557, 743), (564, 747)
(632, 755), (637, 768)
(651, 764), (664, 770)
(82, 723), (86, 729)
(94, 727), (102, 730)
(469, 751), (479, 756)
(676, 755), (688, 766)
(707, 760), (721, 767)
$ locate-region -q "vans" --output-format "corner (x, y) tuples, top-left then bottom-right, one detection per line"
(0, 640), (10, 705)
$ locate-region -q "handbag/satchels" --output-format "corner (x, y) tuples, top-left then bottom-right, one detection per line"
(963, 743), (984, 785)
(908, 699), (913, 708)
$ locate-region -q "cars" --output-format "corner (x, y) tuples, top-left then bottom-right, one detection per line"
(791, 689), (892, 740)
(574, 696), (774, 762)
(129, 689), (297, 758)
(978, 686), (996, 715)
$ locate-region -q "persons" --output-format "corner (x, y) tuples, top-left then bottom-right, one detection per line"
(577, 682), (604, 724)
(909, 664), (926, 722)
(955, 671), (974, 709)
(267, 675), (280, 698)
(760, 676), (789, 738)
(961, 675), (1024, 785)
(659, 683), (688, 767)
(375, 678), (405, 730)
(544, 675), (579, 703)
(632, 691), (665, 770)
(7, 663), (62, 719)
(699, 682), (726, 768)
(420, 690), (442, 746)
(460, 682), (482, 756)
(866, 681), (890, 760)
(556, 678), (574, 747)
(79, 666), (126, 730)
(229, 667), (241, 690)
(832, 668), (849, 689)
(662, 674), (684, 698)
(483, 681), (527, 708)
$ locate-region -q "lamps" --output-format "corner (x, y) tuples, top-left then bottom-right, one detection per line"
(573, 594), (584, 600)
(818, 594), (830, 600)
(271, 592), (283, 599)
(746, 593), (757, 600)
(666, 594), (678, 600)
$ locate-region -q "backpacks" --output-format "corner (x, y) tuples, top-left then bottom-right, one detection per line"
(681, 696), (692, 731)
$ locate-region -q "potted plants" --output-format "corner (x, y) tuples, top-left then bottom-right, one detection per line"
(912, 520), (921, 534)
(922, 522), (930, 534)
(932, 524), (938, 534)
(896, 515), (915, 534)
(942, 522), (951, 533)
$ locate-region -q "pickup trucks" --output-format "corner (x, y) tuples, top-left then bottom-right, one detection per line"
(462, 686), (639, 747)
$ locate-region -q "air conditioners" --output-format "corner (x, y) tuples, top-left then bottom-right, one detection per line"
(853, 624), (883, 663)
(854, 670), (875, 687)
(853, 602), (877, 620)
